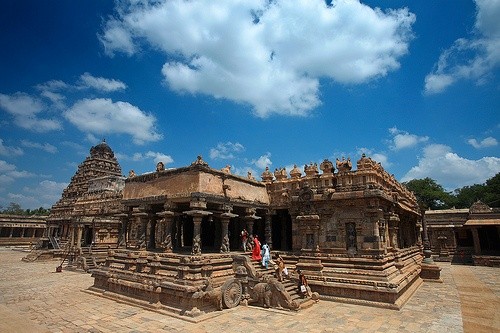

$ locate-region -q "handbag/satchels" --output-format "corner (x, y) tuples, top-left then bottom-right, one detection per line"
(301, 284), (306, 293)
(282, 267), (289, 275)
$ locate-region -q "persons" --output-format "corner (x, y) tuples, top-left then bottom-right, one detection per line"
(297, 269), (309, 299)
(240, 229), (249, 252)
(252, 235), (262, 260)
(261, 243), (271, 269)
(274, 252), (286, 282)
